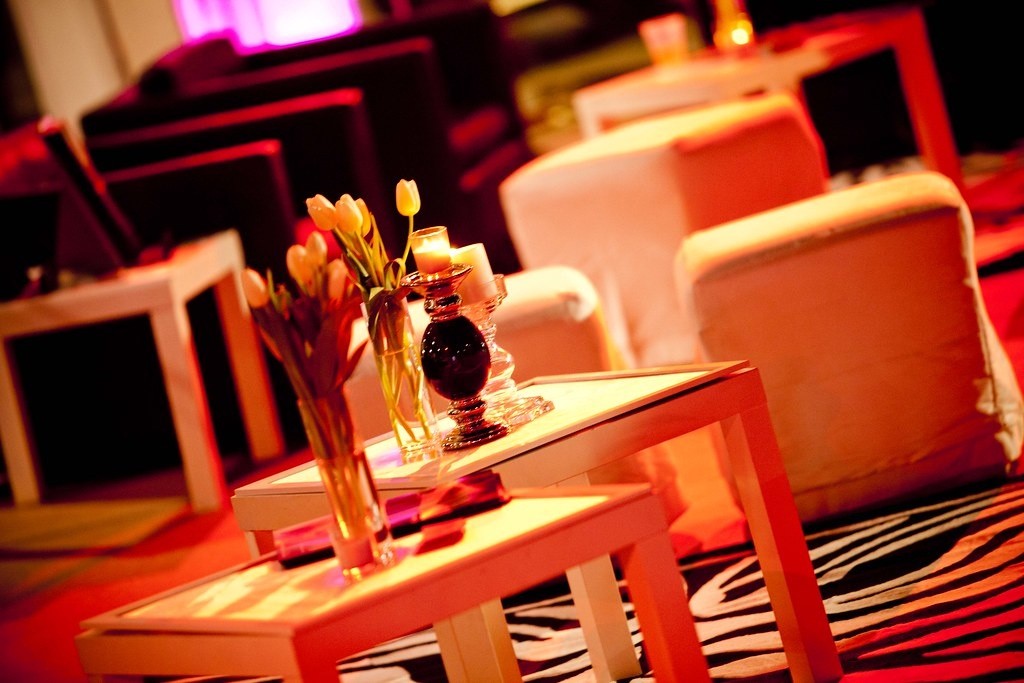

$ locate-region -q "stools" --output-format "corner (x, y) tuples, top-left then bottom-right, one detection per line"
(670, 174), (1024, 523)
(299, 268), (625, 440)
(496, 98), (829, 369)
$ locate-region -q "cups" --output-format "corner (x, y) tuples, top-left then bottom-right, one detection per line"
(637, 13), (692, 68)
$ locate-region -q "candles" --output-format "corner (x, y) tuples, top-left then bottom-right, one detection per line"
(449, 243), (499, 303)
(410, 227), (452, 275)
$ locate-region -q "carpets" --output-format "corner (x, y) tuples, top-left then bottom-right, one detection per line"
(139, 472), (1024, 683)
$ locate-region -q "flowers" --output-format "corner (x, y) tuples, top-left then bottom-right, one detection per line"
(239, 234), (374, 541)
(304, 179), (432, 450)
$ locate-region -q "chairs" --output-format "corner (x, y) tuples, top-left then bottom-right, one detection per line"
(0, 1), (534, 500)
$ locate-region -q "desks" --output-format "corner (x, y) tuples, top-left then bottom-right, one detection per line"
(74, 482), (718, 683)
(1, 228), (286, 511)
(570, 9), (958, 183)
(232, 362), (845, 683)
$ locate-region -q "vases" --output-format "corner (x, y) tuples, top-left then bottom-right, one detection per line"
(296, 390), (395, 579)
(363, 294), (443, 451)
